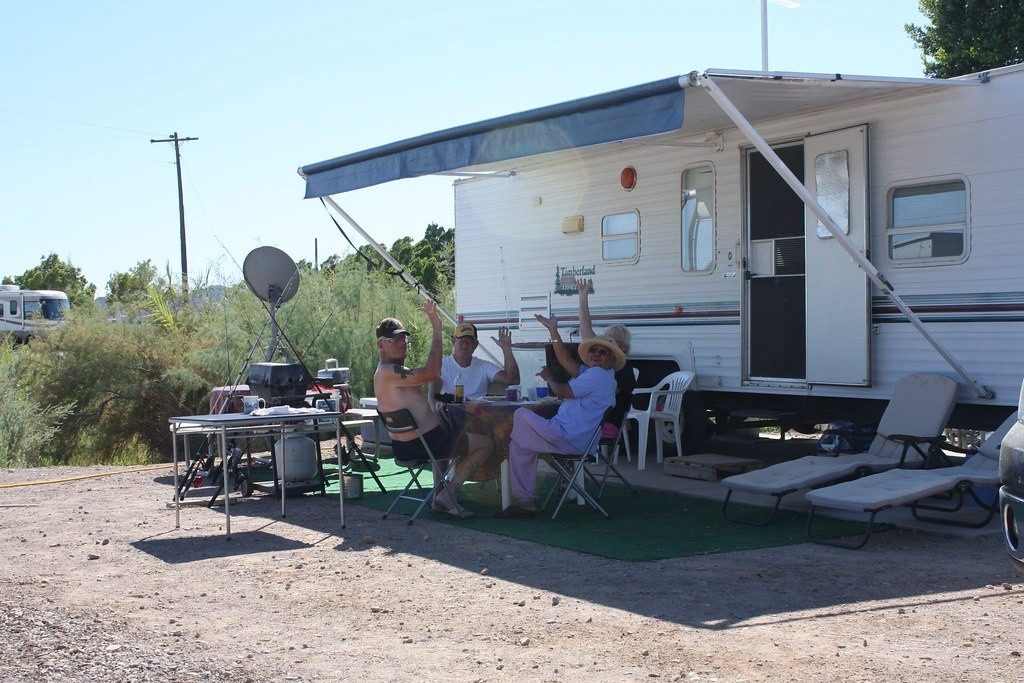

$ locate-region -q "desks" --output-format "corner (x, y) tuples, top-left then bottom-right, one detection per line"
(168, 412), (341, 542)
(435, 402), (563, 512)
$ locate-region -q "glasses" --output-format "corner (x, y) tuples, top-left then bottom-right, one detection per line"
(590, 348), (610, 356)
(382, 335), (405, 343)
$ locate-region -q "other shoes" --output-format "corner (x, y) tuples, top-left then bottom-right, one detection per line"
(565, 488), (577, 501)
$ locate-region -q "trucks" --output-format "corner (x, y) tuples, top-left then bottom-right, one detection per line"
(296, 58), (1024, 448)
(0, 285), (72, 345)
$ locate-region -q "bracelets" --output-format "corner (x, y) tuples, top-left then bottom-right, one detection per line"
(550, 338), (562, 343)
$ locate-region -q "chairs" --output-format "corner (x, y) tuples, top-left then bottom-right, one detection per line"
(538, 406), (612, 522)
(377, 409), (466, 526)
(805, 410), (1018, 550)
(719, 374), (961, 527)
(611, 371), (695, 470)
(567, 394), (633, 500)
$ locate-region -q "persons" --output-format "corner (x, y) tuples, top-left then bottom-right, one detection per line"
(427, 322), (521, 417)
(492, 312), (626, 518)
(373, 299), (494, 520)
(542, 278), (634, 501)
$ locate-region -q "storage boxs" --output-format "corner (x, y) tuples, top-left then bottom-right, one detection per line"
(359, 397), (392, 445)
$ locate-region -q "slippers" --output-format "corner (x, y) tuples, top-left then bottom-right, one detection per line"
(493, 505), (537, 519)
(431, 502), (472, 517)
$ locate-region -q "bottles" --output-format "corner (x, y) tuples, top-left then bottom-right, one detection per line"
(330, 389), (342, 413)
(342, 471), (363, 499)
(454, 372), (464, 403)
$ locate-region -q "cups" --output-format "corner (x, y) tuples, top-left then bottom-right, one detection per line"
(526, 386), (550, 401)
(506, 384), (523, 401)
(243, 395), (266, 414)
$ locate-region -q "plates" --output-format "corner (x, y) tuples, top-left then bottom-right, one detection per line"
(482, 395), (506, 401)
(464, 399), (494, 404)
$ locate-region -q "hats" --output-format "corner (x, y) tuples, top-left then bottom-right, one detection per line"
(376, 318), (410, 340)
(455, 323), (477, 340)
(578, 335), (626, 371)
